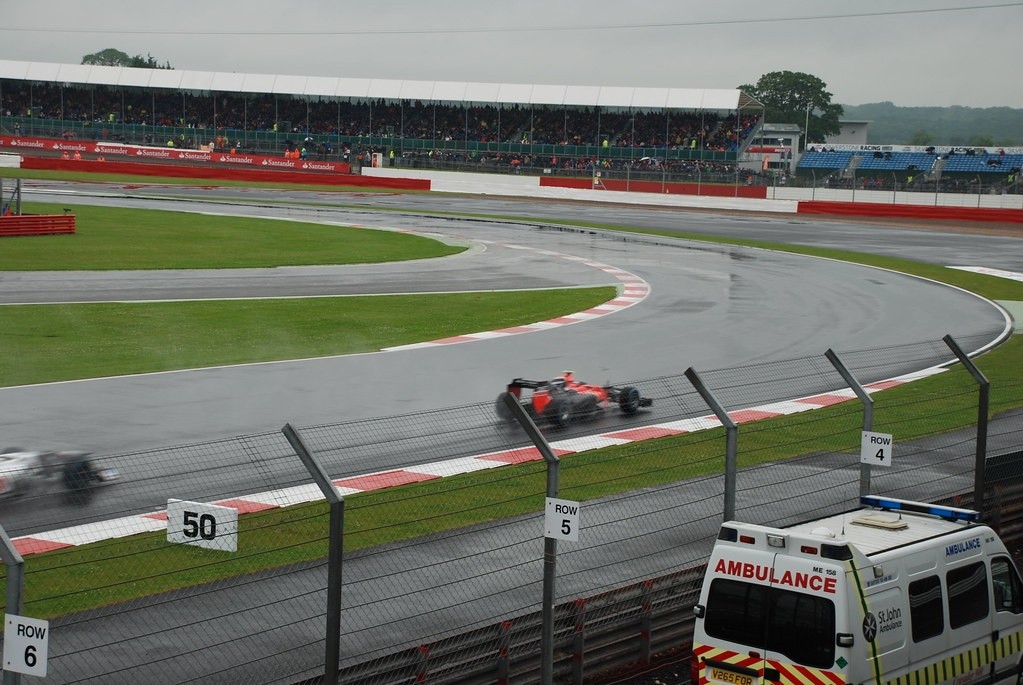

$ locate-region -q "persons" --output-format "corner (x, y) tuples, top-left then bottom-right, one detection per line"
(551, 377), (567, 391)
(0, 78), (1023, 195)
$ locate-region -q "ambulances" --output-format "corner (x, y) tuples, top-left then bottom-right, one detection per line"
(688, 493), (1023, 685)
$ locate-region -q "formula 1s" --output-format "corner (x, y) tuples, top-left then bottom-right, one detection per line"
(495, 369), (653, 428)
(0, 444), (125, 506)
